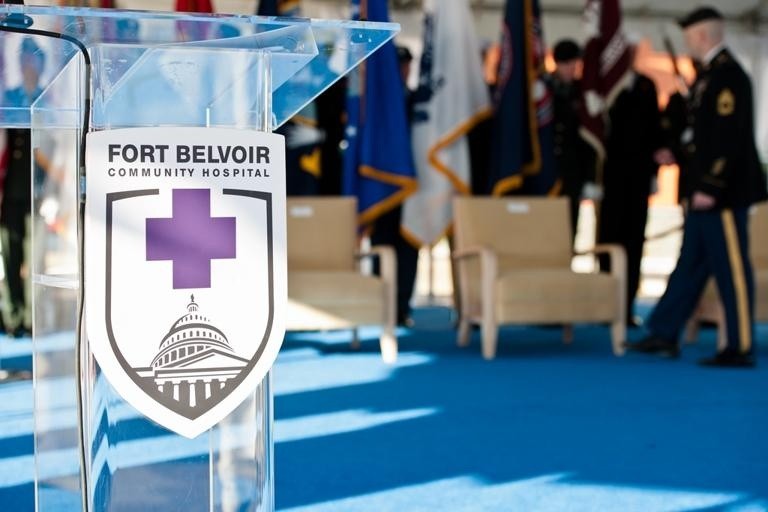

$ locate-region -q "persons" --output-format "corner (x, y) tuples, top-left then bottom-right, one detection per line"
(367, 47), (421, 329)
(539, 37), (592, 341)
(2, 37), (61, 339)
(592, 33), (659, 328)
(622, 7), (765, 367)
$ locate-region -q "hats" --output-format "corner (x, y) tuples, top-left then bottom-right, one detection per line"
(678, 9), (721, 27)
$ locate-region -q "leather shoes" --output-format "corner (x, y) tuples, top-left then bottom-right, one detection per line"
(622, 334), (680, 359)
(698, 347), (755, 368)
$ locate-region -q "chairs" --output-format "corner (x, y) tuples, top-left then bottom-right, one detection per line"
(448, 191), (628, 358)
(685, 204), (768, 353)
(280, 189), (400, 365)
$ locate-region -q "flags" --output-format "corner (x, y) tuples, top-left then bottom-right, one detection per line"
(83, 0), (562, 251)
